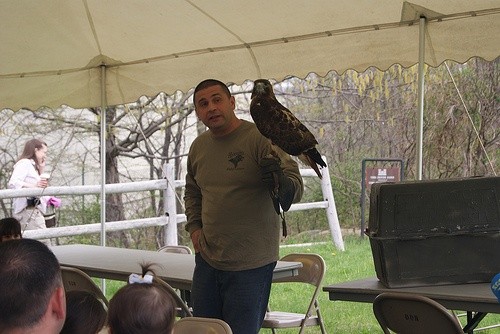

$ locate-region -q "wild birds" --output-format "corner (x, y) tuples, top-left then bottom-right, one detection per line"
(251, 79), (327, 180)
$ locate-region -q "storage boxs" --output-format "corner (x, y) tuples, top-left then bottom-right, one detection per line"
(365, 176), (499, 287)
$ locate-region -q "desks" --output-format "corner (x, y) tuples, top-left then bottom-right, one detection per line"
(322, 277), (500, 315)
(43, 244), (303, 290)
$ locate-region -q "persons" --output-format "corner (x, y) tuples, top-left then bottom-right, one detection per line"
(183, 79), (304, 334)
(6, 138), (52, 247)
(0, 217), (176, 334)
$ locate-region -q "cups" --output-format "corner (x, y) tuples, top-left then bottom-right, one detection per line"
(40, 176), (50, 187)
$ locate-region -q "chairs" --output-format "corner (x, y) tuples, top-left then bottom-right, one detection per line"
(157, 245), (192, 255)
(373, 293), (465, 334)
(260, 253), (328, 334)
(176, 317), (233, 334)
(60, 266), (109, 308)
